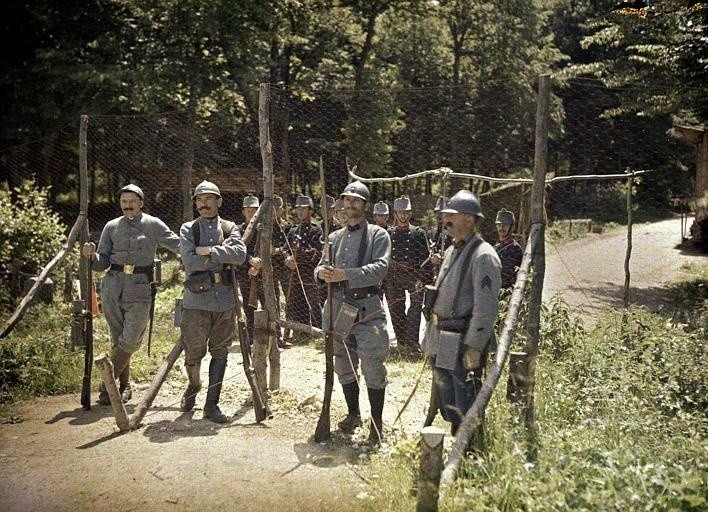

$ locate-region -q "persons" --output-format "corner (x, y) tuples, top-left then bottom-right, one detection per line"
(179, 179), (247, 422)
(372, 200), (391, 231)
(380, 195), (434, 349)
(81, 182), (179, 405)
(491, 207), (523, 290)
(420, 189), (503, 437)
(236, 192), (349, 349)
(421, 195), (454, 324)
(312, 180), (393, 441)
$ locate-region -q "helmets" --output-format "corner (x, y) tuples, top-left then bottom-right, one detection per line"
(340, 181), (371, 202)
(116, 183), (146, 204)
(439, 189), (485, 220)
(191, 180), (222, 199)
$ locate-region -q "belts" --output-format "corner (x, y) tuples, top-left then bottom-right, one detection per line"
(336, 286), (379, 299)
(110, 263), (153, 275)
(210, 271), (231, 283)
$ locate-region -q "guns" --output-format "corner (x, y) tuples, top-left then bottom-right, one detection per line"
(421, 375), (439, 432)
(230, 264), (267, 423)
(283, 222), (304, 342)
(80, 218), (94, 411)
(247, 221), (262, 310)
(312, 243), (336, 443)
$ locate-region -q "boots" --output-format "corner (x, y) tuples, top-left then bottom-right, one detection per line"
(180, 362), (202, 413)
(368, 388), (385, 450)
(98, 344), (133, 406)
(338, 381), (363, 432)
(111, 344), (133, 403)
(203, 358), (228, 423)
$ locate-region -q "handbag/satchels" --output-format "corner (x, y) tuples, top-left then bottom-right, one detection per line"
(434, 329), (461, 371)
(188, 272), (213, 293)
(334, 300), (360, 338)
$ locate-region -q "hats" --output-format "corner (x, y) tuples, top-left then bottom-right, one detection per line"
(495, 210), (516, 225)
(273, 194), (283, 209)
(434, 198), (444, 211)
(294, 195), (314, 210)
(319, 193), (336, 210)
(373, 202), (390, 216)
(242, 195), (260, 208)
(392, 197), (412, 212)
